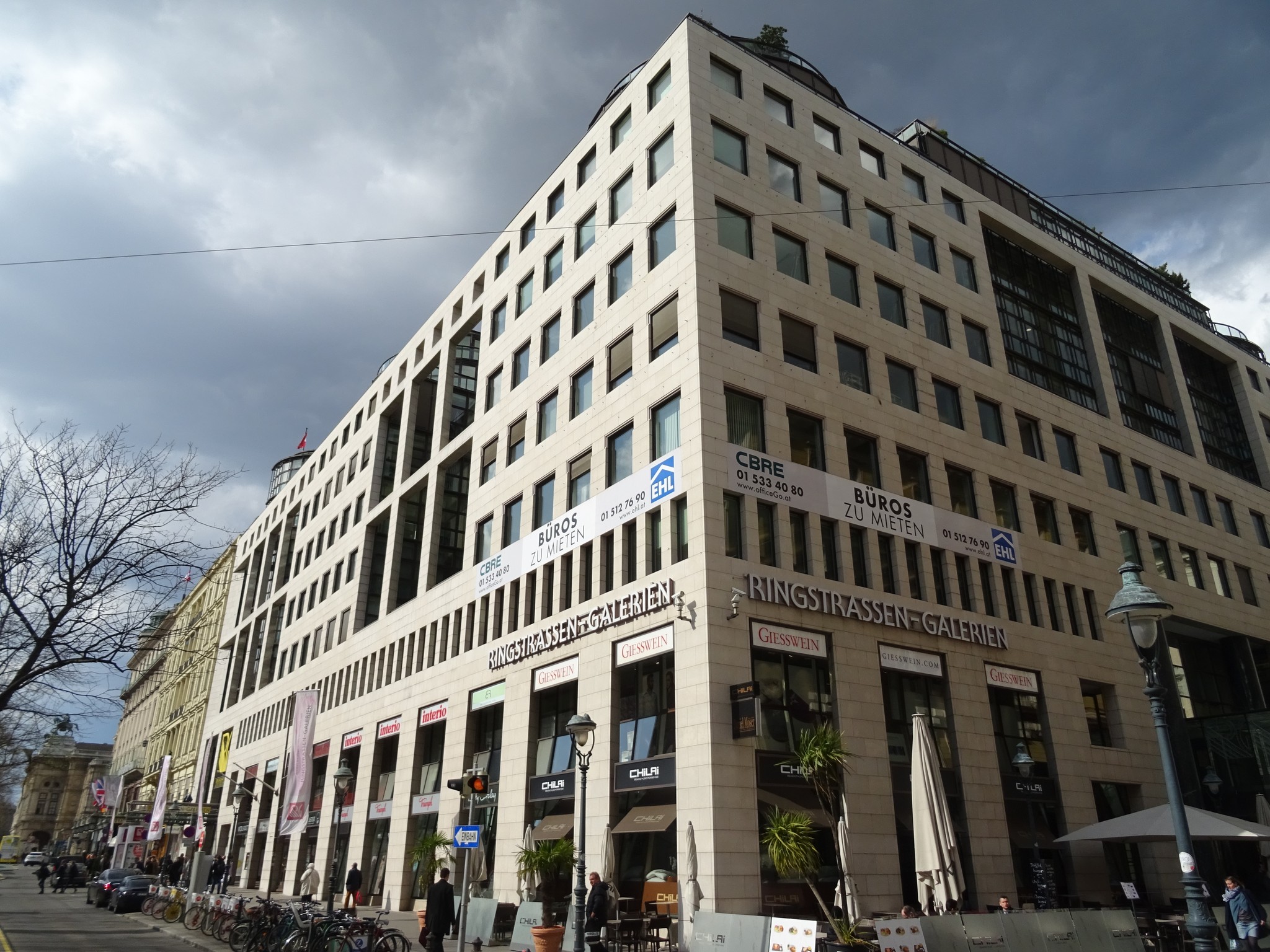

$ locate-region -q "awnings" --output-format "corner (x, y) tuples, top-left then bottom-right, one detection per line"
(109, 835), (117, 846)
(523, 813), (574, 841)
(610, 804), (676, 833)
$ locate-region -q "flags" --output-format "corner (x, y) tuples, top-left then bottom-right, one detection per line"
(297, 432), (307, 449)
(91, 777), (113, 812)
(182, 569), (190, 581)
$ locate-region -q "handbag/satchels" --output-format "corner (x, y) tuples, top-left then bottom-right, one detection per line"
(356, 889), (363, 903)
(1229, 939), (1243, 951)
(418, 925), (430, 949)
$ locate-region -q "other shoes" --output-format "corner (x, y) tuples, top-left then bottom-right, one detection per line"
(39, 888), (77, 894)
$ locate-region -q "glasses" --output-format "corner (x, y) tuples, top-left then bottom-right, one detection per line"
(351, 865), (354, 866)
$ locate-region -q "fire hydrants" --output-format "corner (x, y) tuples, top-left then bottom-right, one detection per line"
(471, 936), (483, 952)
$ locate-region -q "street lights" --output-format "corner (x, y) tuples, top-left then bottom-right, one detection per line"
(1101, 558), (1228, 952)
(565, 714), (597, 952)
(326, 757), (354, 914)
(1011, 741), (1053, 906)
(159, 800), (180, 884)
(49, 815), (110, 881)
(220, 782), (246, 896)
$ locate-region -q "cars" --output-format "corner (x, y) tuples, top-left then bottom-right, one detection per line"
(85, 867), (137, 908)
(107, 875), (162, 915)
(54, 864), (86, 888)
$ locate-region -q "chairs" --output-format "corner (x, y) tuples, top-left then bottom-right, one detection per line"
(807, 897), (1197, 952)
(598, 897), (678, 952)
(493, 902), (519, 941)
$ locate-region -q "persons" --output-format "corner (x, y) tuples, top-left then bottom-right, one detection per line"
(942, 898), (959, 916)
(1222, 876), (1270, 952)
(128, 853), (226, 895)
(32, 859), (78, 894)
(344, 862), (363, 909)
(299, 863), (320, 903)
(86, 853), (113, 881)
(585, 871), (607, 951)
(423, 867), (457, 952)
(901, 905), (927, 918)
(996, 895), (1018, 913)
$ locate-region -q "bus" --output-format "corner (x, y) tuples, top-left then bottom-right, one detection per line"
(0, 835), (21, 863)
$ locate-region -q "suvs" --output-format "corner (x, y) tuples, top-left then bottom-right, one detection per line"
(24, 851), (49, 866)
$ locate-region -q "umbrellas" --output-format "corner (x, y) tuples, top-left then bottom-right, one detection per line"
(468, 825), (487, 897)
(685, 820), (704, 924)
(516, 824), (542, 902)
(911, 711), (967, 917)
(601, 823), (620, 913)
(833, 817), (863, 925)
(1053, 791), (1270, 857)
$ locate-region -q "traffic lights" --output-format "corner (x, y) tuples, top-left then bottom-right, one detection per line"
(466, 774), (489, 794)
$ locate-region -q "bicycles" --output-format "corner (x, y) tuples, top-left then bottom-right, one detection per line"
(141, 883), (412, 952)
